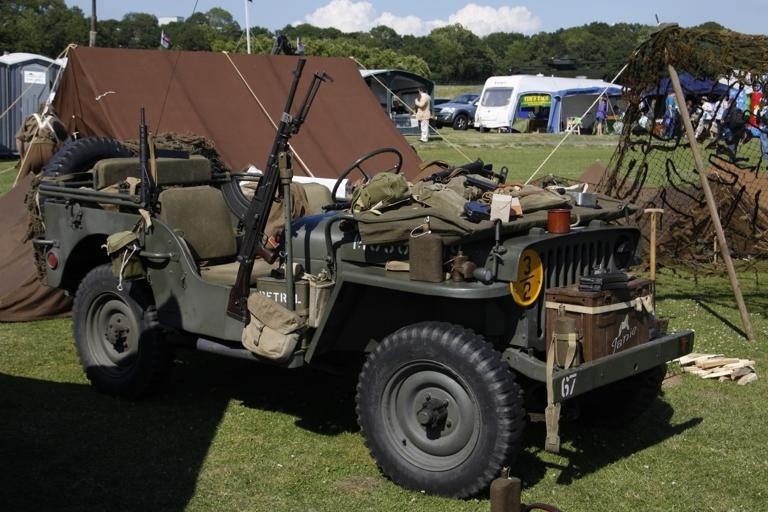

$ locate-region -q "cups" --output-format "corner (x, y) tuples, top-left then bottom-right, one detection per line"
(547, 209), (580, 234)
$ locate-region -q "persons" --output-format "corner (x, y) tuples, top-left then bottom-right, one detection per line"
(612, 104), (626, 134)
(15, 100), (56, 180)
(593, 97), (609, 135)
(631, 91), (749, 164)
(414, 86), (432, 145)
(390, 101), (411, 128)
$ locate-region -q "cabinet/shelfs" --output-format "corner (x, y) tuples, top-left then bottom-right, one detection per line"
(565, 117), (582, 137)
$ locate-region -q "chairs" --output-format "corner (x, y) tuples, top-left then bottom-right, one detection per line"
(95, 153), (287, 285)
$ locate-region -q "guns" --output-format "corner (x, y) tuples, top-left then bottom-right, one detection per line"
(221, 60), (334, 324)
(133, 107), (161, 247)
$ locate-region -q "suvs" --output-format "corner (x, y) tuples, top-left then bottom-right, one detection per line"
(30, 56), (706, 498)
(432, 92), (483, 132)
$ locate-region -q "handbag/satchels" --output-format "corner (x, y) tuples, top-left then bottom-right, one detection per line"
(240, 292), (306, 364)
(349, 172), (412, 215)
